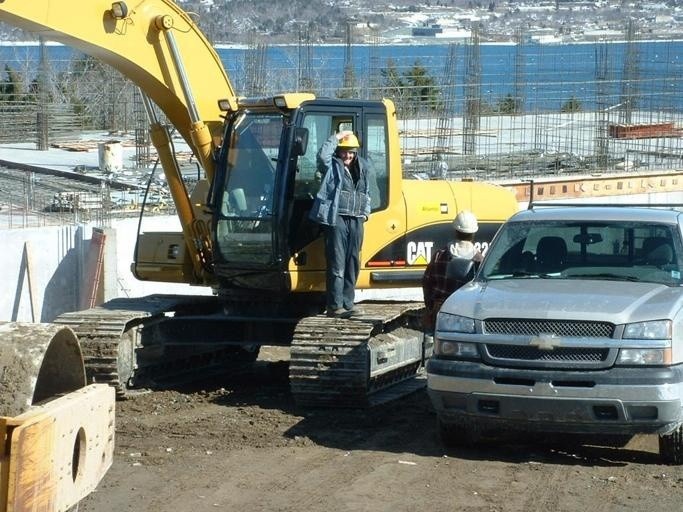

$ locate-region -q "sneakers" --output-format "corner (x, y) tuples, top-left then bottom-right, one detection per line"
(327, 306), (364, 318)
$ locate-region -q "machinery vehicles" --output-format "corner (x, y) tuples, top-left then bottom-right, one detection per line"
(0, 2), (522, 415)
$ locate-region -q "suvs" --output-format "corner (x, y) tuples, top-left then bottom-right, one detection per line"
(425, 201), (682, 463)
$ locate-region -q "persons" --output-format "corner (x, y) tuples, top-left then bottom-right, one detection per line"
(423, 210), (484, 338)
(309, 130), (373, 320)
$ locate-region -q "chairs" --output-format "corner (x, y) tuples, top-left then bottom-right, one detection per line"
(535, 237), (674, 273)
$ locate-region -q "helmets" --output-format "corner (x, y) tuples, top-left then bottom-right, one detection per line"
(337, 134), (360, 148)
(452, 209), (479, 234)
